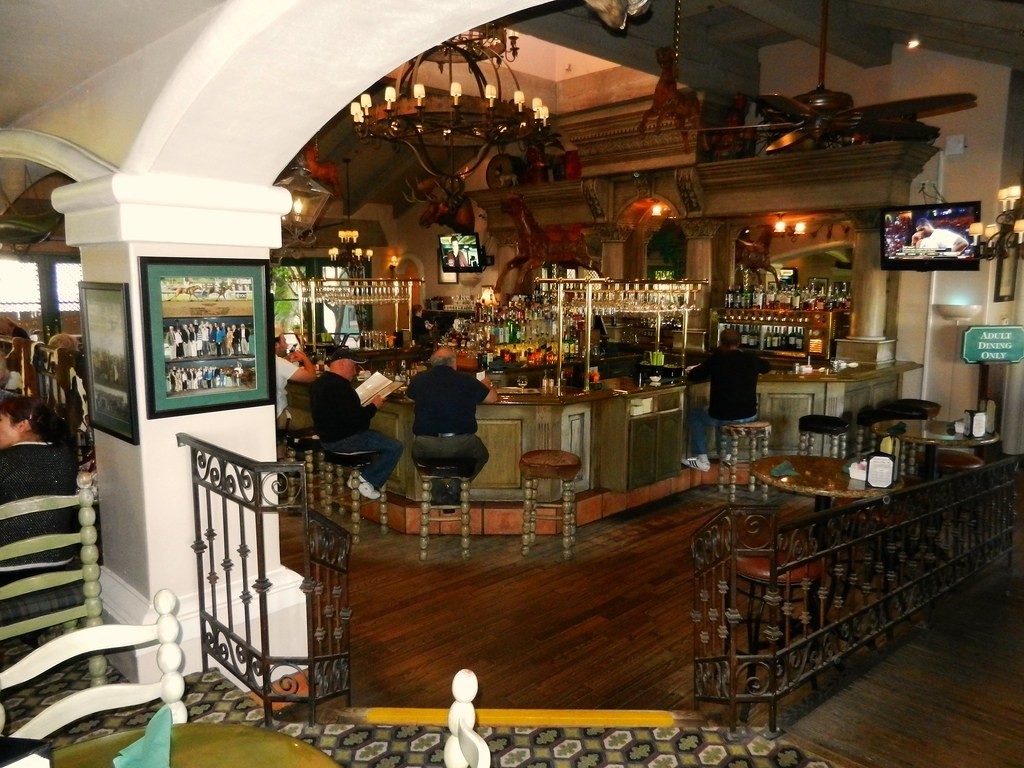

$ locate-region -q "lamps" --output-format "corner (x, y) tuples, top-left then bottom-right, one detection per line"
(391, 256), (399, 270)
(794, 220), (806, 234)
(273, 157), (331, 255)
(350, 23), (551, 205)
(934, 304), (982, 323)
(774, 213), (786, 233)
(652, 201), (661, 216)
(328, 215), (374, 263)
(969, 184), (1024, 256)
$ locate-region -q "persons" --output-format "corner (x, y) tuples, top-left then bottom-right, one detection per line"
(409, 352), (498, 514)
(448, 237), (468, 266)
(169, 366), (240, 392)
(309, 355), (403, 499)
(1, 351), (82, 646)
(167, 322), (250, 360)
(275, 324), (319, 453)
(413, 305), (429, 343)
(911, 218), (969, 255)
(681, 329), (771, 472)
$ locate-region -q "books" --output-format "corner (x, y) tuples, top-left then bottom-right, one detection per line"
(355, 372), (404, 406)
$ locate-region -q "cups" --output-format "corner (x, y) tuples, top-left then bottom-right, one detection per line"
(301, 278), (410, 305)
(317, 348), (326, 371)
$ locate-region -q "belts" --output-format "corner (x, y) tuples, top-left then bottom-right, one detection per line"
(425, 431), (465, 438)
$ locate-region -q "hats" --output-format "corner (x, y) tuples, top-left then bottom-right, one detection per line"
(323, 347), (358, 364)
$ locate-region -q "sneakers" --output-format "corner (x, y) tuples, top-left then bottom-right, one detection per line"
(346, 474), (367, 490)
(682, 456), (711, 472)
(357, 480), (381, 500)
(722, 453), (731, 467)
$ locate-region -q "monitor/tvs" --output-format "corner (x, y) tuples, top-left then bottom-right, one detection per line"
(880, 200), (982, 270)
(438, 232), (483, 273)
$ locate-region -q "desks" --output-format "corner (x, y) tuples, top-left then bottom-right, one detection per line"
(752, 454), (903, 619)
(49, 716), (349, 768)
(870, 420), (1000, 567)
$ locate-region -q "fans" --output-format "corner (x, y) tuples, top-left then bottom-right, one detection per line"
(678, 0), (978, 153)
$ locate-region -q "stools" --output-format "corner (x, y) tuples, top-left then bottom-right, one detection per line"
(896, 398), (940, 476)
(522, 449), (580, 555)
(856, 413), (903, 472)
(884, 407), (931, 475)
(723, 541), (827, 720)
(418, 457), (479, 559)
(798, 415), (850, 457)
(323, 448), (390, 538)
(823, 498), (916, 639)
(917, 447), (982, 543)
(283, 428), (322, 509)
(716, 422), (773, 500)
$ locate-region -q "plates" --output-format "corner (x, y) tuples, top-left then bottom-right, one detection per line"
(486, 153), (516, 189)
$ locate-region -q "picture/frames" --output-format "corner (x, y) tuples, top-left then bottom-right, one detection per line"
(994, 223), (1020, 302)
(77, 283), (138, 445)
(809, 278), (828, 290)
(138, 252), (274, 418)
(437, 248), (459, 284)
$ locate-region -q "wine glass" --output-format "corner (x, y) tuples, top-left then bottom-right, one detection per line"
(517, 375), (528, 394)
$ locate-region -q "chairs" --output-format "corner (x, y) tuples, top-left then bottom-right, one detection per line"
(0, 471), (107, 688)
(1, 590), (188, 745)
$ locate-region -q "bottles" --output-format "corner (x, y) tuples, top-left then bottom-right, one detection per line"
(360, 330), (387, 351)
(460, 294), (579, 365)
(549, 369), (554, 394)
(541, 370), (549, 396)
(383, 360), (430, 388)
(724, 284), (838, 352)
